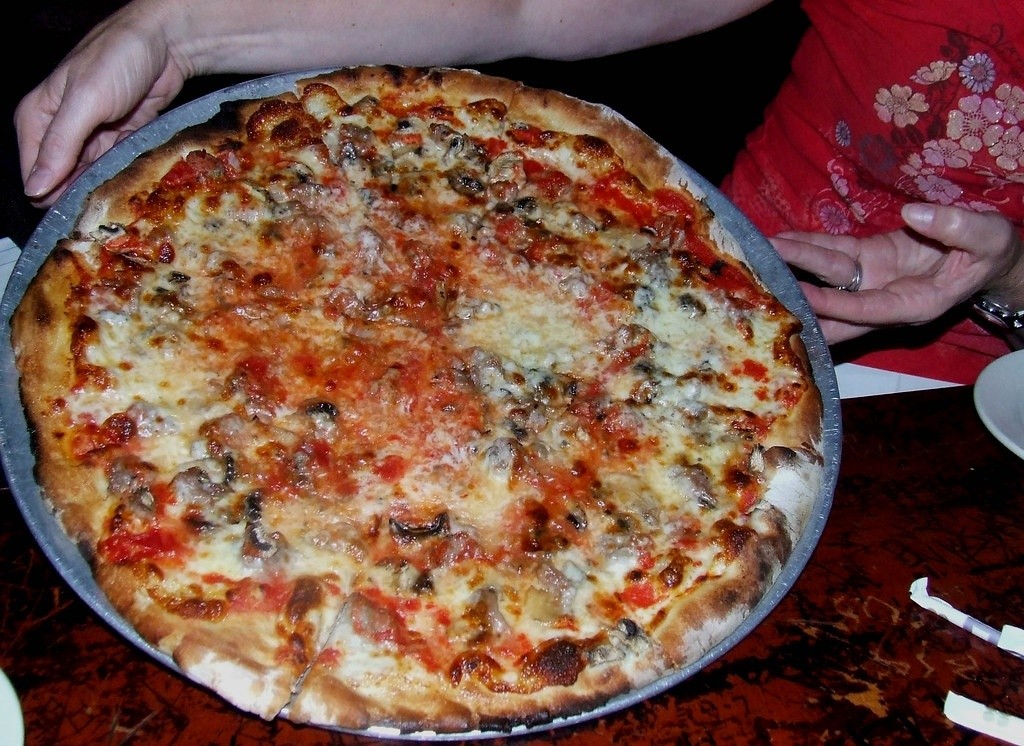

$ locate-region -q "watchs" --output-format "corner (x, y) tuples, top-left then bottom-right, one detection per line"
(972, 297), (1024, 329)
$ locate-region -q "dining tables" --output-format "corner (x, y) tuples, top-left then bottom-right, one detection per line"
(1, 383), (1024, 745)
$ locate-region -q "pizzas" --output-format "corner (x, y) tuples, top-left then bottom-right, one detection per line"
(11, 61), (822, 731)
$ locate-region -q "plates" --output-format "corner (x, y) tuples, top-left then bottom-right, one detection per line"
(974, 346), (1024, 459)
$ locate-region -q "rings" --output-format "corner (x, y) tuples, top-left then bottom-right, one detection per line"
(835, 260), (864, 292)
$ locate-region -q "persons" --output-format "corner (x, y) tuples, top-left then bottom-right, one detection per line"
(12, 0), (1024, 401)
